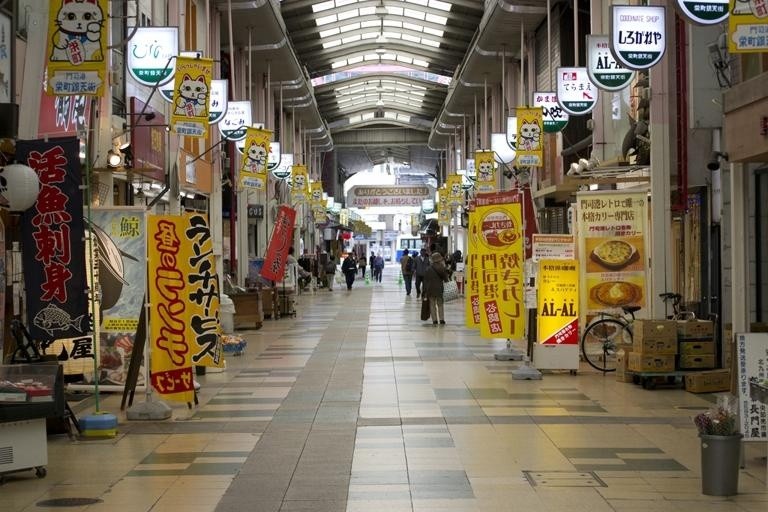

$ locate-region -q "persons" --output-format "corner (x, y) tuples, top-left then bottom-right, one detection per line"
(286, 246), (461, 323)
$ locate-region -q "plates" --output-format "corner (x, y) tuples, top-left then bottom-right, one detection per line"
(623, 325), (633, 345)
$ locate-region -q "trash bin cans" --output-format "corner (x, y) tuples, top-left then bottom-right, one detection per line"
(220, 294), (236, 334)
(697, 432), (744, 495)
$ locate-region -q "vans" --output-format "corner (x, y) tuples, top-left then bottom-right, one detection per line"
(371, 245), (390, 260)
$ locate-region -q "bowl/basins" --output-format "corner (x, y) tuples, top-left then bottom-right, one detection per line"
(587, 314), (620, 341)
(593, 239), (637, 267)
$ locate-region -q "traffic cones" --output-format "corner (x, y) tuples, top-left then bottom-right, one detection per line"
(364, 271), (371, 286)
(397, 270), (404, 286)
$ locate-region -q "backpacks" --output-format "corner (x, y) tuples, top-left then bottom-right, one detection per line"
(404, 256), (414, 273)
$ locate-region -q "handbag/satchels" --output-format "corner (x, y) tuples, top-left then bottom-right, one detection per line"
(442, 277), (459, 301)
(421, 297), (431, 320)
(431, 265), (448, 279)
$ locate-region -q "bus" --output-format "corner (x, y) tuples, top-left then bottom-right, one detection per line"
(396, 233), (425, 265)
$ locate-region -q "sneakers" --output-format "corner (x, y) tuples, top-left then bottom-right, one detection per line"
(416, 289), (422, 299)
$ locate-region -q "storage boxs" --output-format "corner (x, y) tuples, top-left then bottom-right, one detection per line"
(613, 316), (736, 395)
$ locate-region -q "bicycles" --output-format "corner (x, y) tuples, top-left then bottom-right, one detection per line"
(579, 291), (682, 378)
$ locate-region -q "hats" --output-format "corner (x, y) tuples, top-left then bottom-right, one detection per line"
(430, 252), (444, 264)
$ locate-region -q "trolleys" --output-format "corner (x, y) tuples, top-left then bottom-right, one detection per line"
(275, 270), (300, 319)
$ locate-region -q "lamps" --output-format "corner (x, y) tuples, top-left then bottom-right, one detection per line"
(566, 153), (600, 178)
(707, 150), (729, 171)
(107, 111), (173, 168)
(502, 164), (531, 180)
